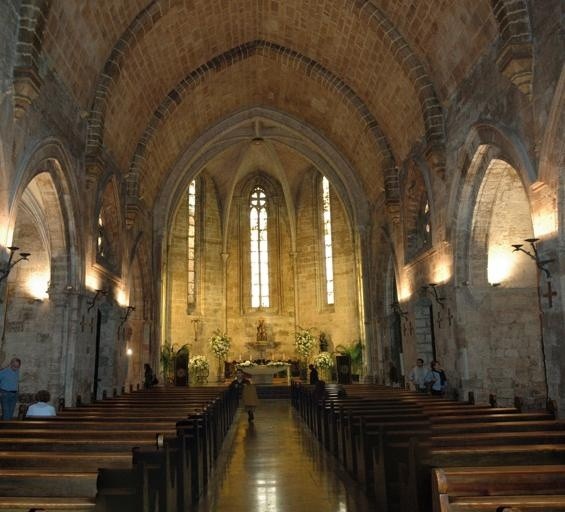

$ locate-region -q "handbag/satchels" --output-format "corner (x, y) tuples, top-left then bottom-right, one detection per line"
(426, 382), (432, 390)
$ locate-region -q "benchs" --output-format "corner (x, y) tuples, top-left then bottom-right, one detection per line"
(0, 385), (240, 511)
(290, 382), (565, 510)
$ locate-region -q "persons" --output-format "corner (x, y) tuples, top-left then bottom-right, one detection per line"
(424, 361), (448, 396)
(225, 368), (233, 378)
(389, 363), (397, 384)
(25, 390), (56, 416)
(0, 358), (21, 420)
(291, 366), (307, 380)
(408, 358), (429, 393)
(308, 364), (318, 384)
(241, 380), (259, 421)
(143, 364), (155, 390)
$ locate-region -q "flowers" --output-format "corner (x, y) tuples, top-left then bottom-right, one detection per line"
(208, 329), (231, 358)
(188, 354), (210, 372)
(294, 332), (315, 356)
(313, 351), (335, 369)
